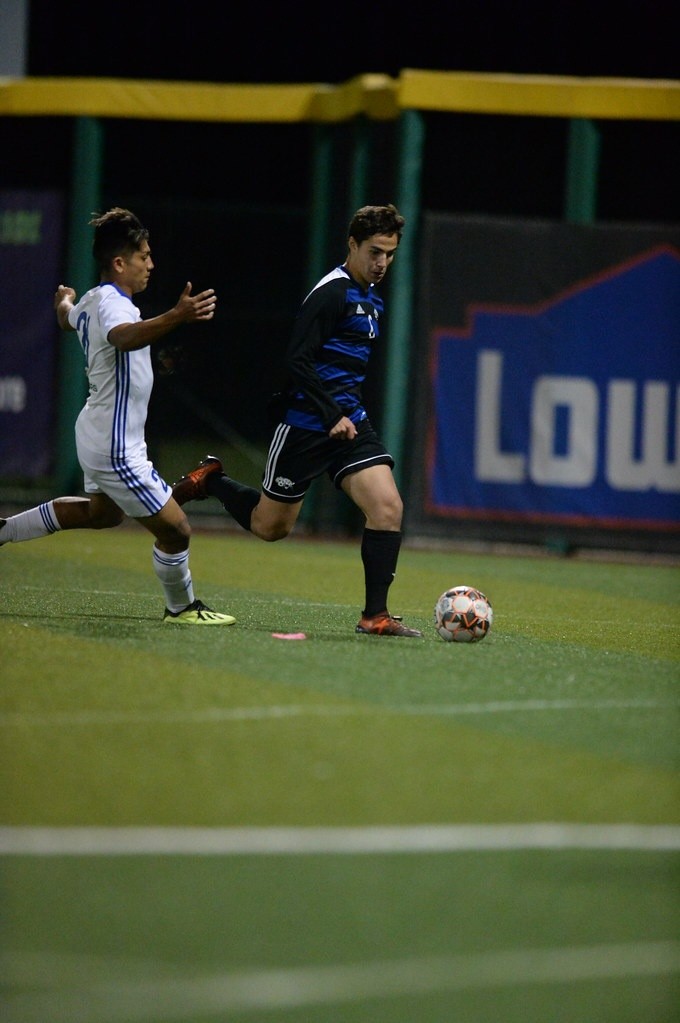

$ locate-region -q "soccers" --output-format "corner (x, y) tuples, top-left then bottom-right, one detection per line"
(434, 585), (494, 643)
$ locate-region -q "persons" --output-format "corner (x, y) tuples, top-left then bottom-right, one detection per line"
(172, 204), (424, 638)
(0, 209), (237, 626)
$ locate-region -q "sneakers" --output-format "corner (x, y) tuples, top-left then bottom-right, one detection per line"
(171, 455), (224, 506)
(164, 598), (236, 626)
(355, 610), (421, 637)
